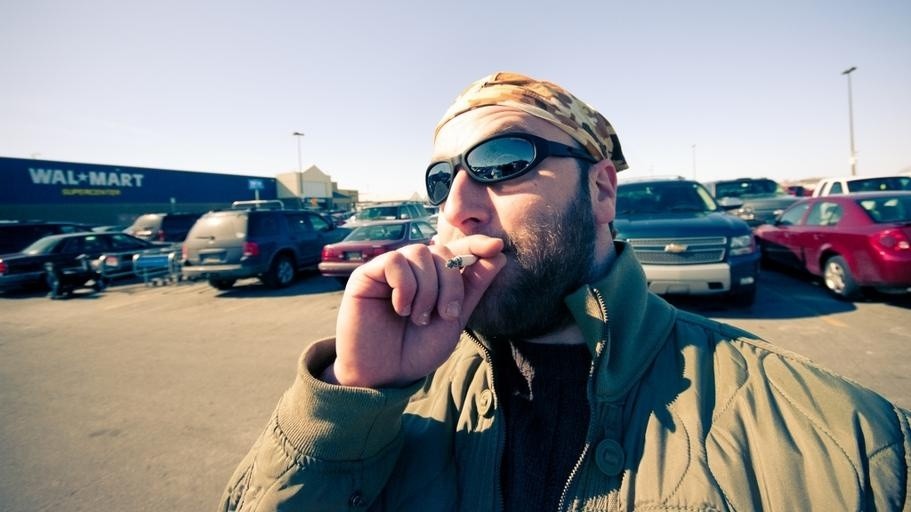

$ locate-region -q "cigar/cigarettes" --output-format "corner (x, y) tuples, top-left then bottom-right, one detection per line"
(444, 254), (482, 269)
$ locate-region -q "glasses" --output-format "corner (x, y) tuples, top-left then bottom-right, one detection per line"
(422, 130), (601, 207)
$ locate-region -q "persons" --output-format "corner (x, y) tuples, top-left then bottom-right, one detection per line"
(212, 69), (911, 511)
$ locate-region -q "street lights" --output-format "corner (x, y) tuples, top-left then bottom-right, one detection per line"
(293, 132), (304, 171)
(842, 66), (857, 176)
